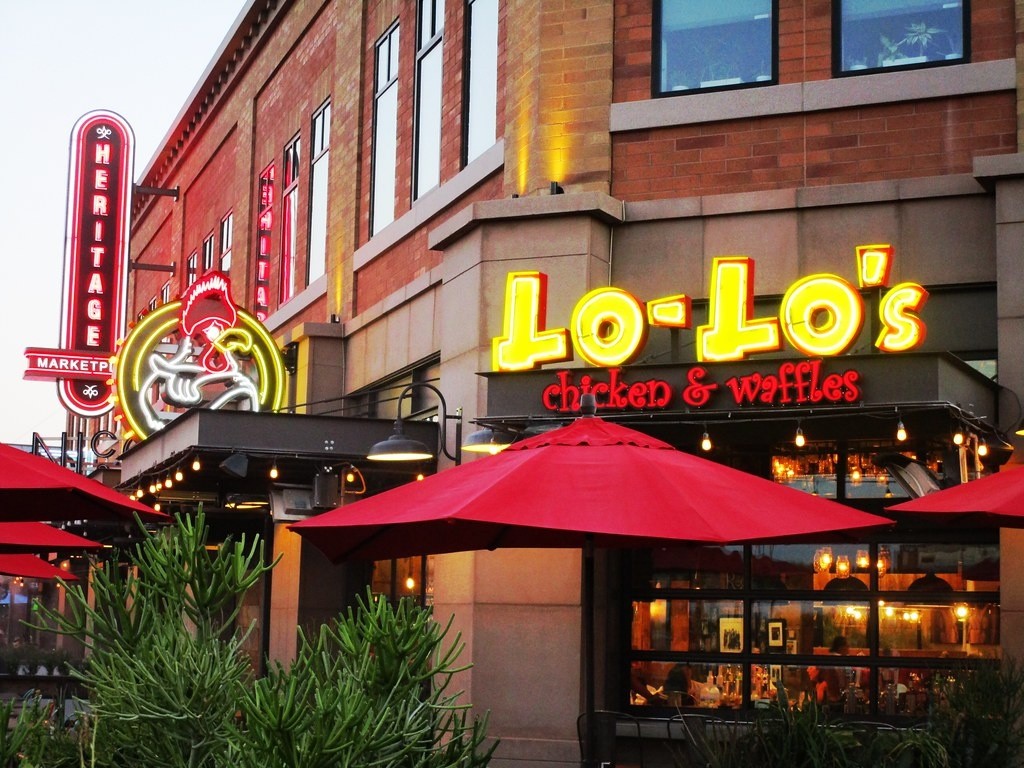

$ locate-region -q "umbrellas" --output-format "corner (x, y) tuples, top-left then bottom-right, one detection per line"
(0, 521), (102, 554)
(290, 392), (897, 710)
(885, 465), (1024, 529)
(0, 443), (168, 522)
(0, 554), (80, 581)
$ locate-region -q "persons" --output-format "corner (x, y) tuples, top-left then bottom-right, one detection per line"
(630, 670), (668, 705)
(818, 636), (853, 713)
(658, 663), (704, 693)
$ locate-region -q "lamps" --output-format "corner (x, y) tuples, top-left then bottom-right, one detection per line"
(551, 180), (563, 194)
(749, 575), (792, 606)
(330, 314), (339, 323)
(367, 383), (462, 469)
(651, 574), (672, 650)
(460, 423), (522, 455)
(772, 449), (938, 485)
(217, 451), (248, 478)
(814, 545), (971, 624)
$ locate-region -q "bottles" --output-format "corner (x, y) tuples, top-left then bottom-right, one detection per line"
(706, 664), (744, 700)
(885, 683), (894, 714)
(754, 666), (780, 699)
(906, 676), (918, 713)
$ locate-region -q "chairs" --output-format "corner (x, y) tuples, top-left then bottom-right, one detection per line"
(578, 711), (906, 768)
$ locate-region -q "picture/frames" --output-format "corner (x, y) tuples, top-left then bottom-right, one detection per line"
(717, 614), (743, 653)
(766, 619), (787, 651)
